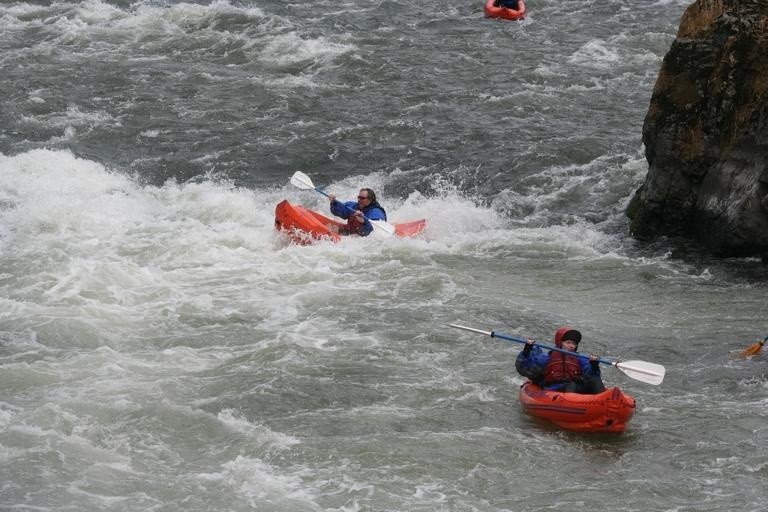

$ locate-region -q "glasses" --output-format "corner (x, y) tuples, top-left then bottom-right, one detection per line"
(357, 195), (370, 201)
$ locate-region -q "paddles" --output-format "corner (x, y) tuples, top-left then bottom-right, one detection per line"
(289, 171), (394, 237)
(740, 335), (768, 357)
(447, 323), (665, 386)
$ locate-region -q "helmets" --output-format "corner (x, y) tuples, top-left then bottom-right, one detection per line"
(555, 326), (582, 348)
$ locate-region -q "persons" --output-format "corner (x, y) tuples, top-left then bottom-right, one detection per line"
(515, 323), (606, 397)
(327, 187), (388, 238)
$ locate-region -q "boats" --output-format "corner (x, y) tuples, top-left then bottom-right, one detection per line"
(274, 199), (427, 246)
(519, 379), (636, 433)
(484, 0), (526, 21)
